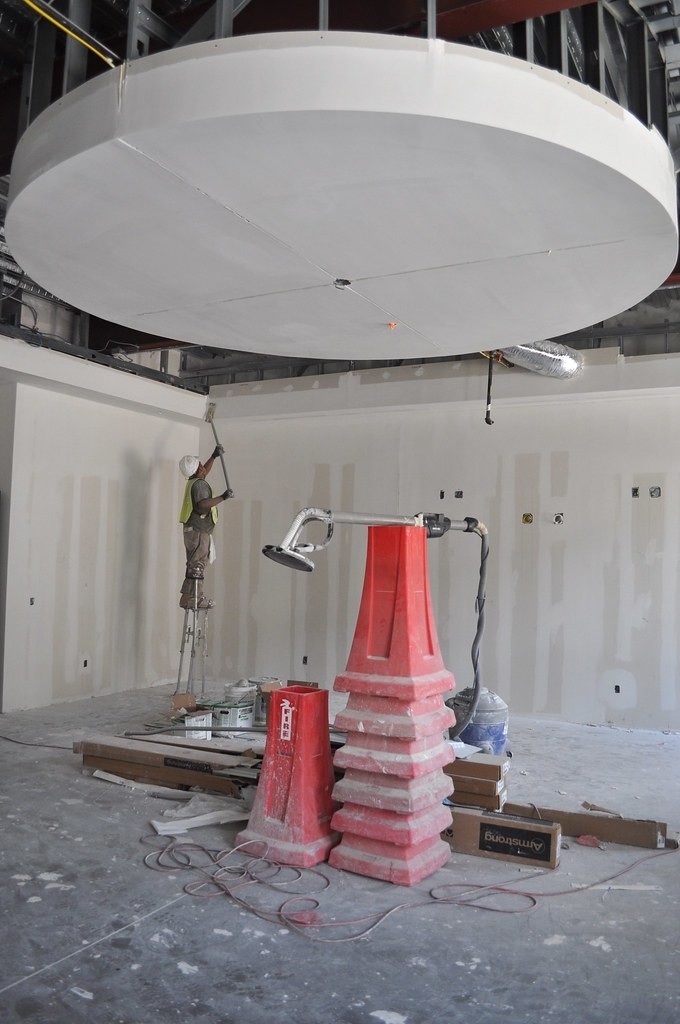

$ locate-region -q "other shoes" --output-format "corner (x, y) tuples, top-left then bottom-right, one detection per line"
(180, 592), (216, 609)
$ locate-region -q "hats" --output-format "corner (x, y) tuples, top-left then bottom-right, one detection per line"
(179, 455), (200, 477)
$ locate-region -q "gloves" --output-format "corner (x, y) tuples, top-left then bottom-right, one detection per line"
(221, 489), (234, 500)
(212, 444), (226, 459)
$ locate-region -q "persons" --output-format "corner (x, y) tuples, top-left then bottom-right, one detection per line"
(178, 443), (235, 610)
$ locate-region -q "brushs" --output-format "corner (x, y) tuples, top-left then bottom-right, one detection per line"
(204, 402), (234, 499)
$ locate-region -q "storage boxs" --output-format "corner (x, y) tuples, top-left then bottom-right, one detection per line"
(448, 788), (507, 811)
(440, 807), (562, 869)
(73, 735), (262, 784)
(196, 700), (254, 737)
(168, 693), (220, 742)
(446, 774), (506, 798)
(258, 680), (318, 727)
(116, 734), (265, 761)
(502, 799), (668, 850)
(81, 754), (245, 800)
(442, 753), (511, 782)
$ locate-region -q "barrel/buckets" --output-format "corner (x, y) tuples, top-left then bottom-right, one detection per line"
(224, 683), (256, 723)
(248, 677), (280, 726)
